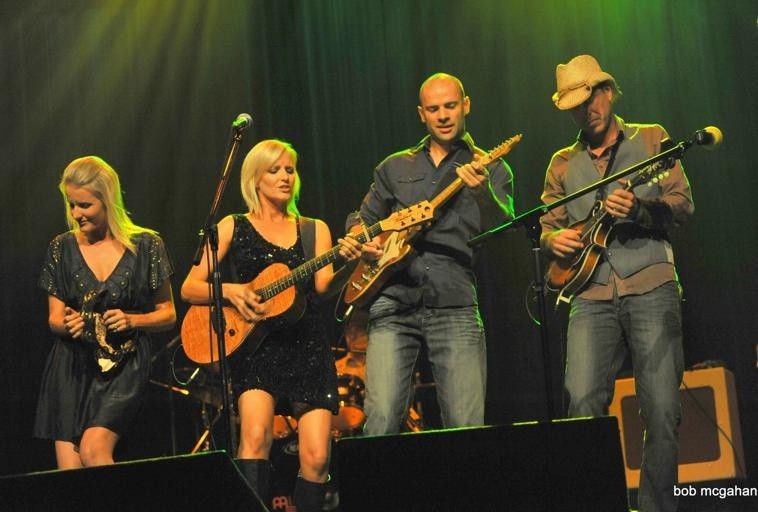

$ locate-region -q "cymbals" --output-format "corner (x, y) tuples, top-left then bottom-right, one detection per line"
(331, 346), (367, 354)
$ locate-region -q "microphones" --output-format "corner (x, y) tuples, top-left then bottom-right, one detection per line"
(692, 125), (724, 147)
(228, 111), (253, 129)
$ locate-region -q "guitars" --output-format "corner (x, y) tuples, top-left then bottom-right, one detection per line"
(542, 157), (674, 308)
(181, 201), (433, 363)
(344, 134), (525, 307)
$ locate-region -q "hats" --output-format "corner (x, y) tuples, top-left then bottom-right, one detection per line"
(552, 55), (622, 110)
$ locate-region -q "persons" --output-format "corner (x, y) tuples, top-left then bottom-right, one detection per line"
(180, 135), (363, 512)
(32, 154), (179, 470)
(344, 70), (516, 436)
(537, 51), (697, 512)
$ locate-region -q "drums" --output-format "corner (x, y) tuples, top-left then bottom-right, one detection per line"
(269, 398), (297, 439)
(331, 373), (366, 432)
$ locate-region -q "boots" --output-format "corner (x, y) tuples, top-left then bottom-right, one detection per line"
(295, 470), (331, 512)
(234, 459), (268, 505)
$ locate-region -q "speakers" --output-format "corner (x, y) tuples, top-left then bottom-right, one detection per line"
(0, 448), (267, 512)
(337, 412), (628, 511)
(606, 366), (747, 491)
